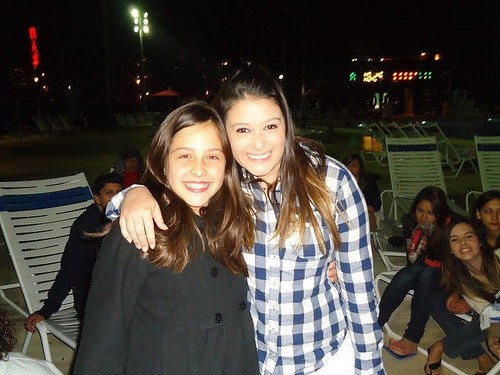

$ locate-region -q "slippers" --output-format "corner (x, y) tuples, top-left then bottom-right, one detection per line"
(424, 348), (441, 375)
(383, 345), (417, 359)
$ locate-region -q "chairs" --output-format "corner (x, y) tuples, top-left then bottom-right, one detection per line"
(32, 112), (160, 131)
(0, 172), (95, 366)
(359, 118), (500, 375)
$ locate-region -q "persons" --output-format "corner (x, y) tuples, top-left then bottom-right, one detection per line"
(69, 61), (392, 375)
(0, 143), (500, 375)
